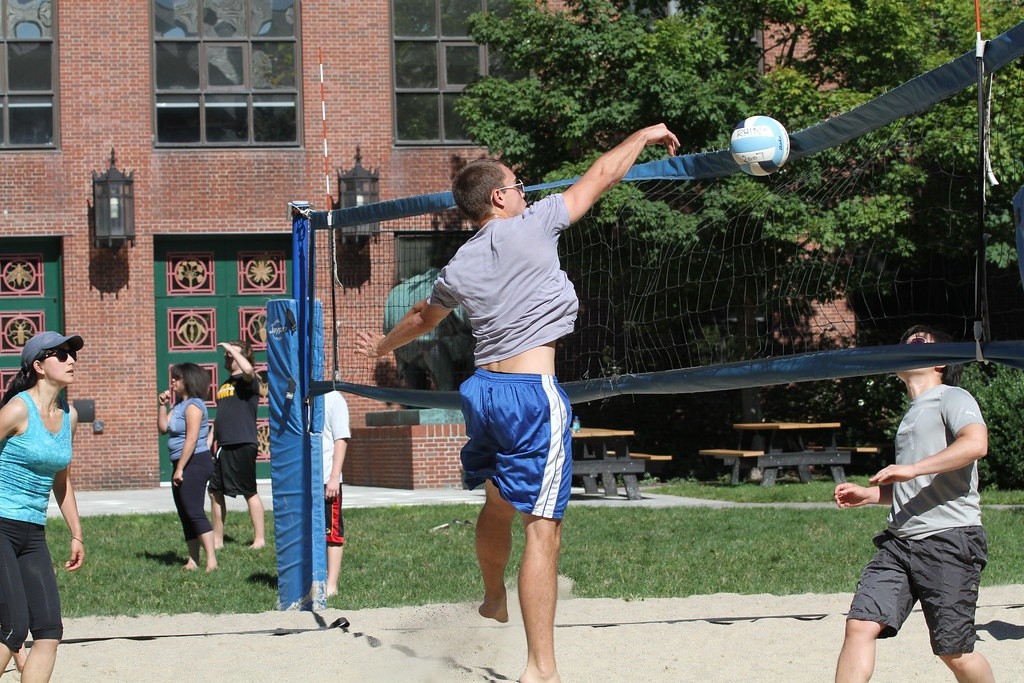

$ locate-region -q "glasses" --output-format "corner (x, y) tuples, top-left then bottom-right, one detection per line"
(488, 178), (524, 203)
(171, 373), (182, 381)
(905, 335), (927, 346)
(39, 348), (77, 363)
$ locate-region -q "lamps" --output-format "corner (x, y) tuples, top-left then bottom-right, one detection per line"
(89, 147), (135, 255)
(336, 146), (381, 253)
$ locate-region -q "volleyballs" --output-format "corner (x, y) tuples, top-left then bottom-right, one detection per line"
(730, 115), (791, 177)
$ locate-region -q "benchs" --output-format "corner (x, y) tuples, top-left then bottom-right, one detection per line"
(698, 446), (878, 465)
(604, 451), (672, 461)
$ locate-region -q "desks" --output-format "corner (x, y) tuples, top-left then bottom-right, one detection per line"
(570, 427), (641, 500)
(731, 423), (846, 486)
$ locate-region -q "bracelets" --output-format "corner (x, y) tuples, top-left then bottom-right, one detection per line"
(71, 536), (83, 544)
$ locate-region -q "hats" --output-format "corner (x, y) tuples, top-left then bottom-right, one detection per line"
(21, 331), (84, 369)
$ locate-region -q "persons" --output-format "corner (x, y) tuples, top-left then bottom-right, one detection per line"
(0, 330), (85, 683)
(208, 340), (265, 550)
(322, 390), (351, 596)
(834, 323), (995, 683)
(353, 122), (680, 683)
(157, 362), (219, 573)
